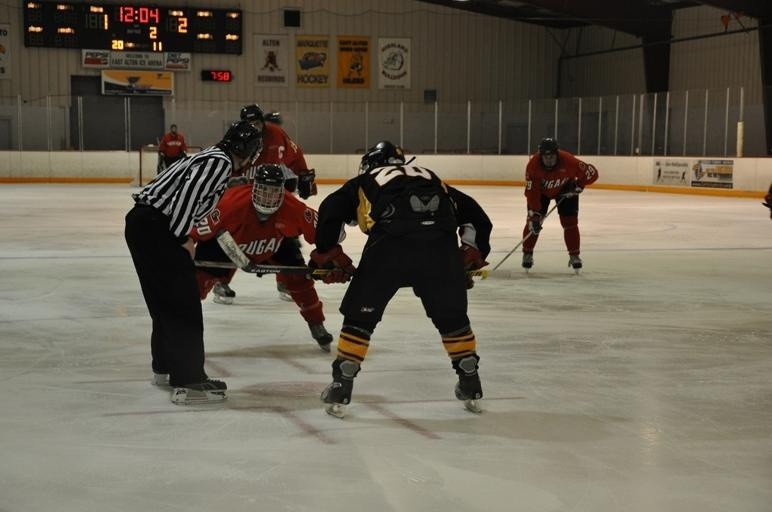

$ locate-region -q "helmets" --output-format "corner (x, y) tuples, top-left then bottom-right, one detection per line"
(220, 103), (266, 162)
(252, 164), (286, 223)
(358, 141), (405, 175)
(538, 136), (560, 173)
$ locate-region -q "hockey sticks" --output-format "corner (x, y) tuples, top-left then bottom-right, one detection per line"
(481, 196), (563, 282)
(212, 226), (490, 278)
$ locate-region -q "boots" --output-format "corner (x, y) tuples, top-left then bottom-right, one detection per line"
(453, 355), (483, 400)
(321, 358), (360, 404)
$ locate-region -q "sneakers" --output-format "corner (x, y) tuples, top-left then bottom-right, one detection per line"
(568, 254), (583, 267)
(308, 320), (333, 344)
(213, 281), (235, 297)
(174, 380), (228, 393)
(522, 249), (534, 267)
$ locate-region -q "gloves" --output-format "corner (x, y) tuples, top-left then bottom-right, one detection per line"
(561, 182), (583, 198)
(297, 169), (316, 199)
(308, 244), (357, 284)
(459, 243), (488, 290)
(526, 209), (545, 234)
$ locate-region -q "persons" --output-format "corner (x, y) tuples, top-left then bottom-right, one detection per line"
(123, 121), (264, 405)
(305, 140), (494, 418)
(522, 137), (599, 277)
(210, 103), (318, 306)
(264, 111), (282, 127)
(182, 163), (347, 352)
(157, 124), (188, 174)
